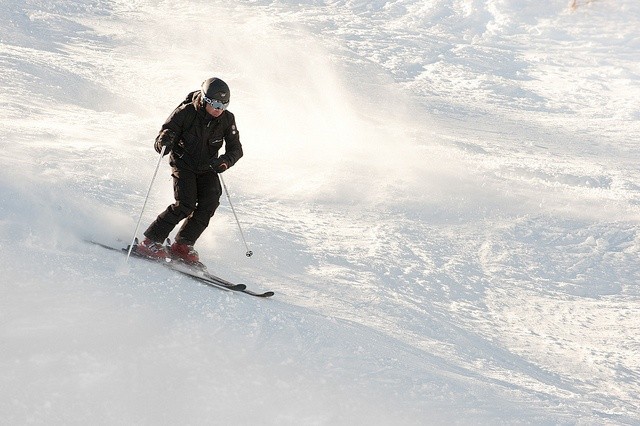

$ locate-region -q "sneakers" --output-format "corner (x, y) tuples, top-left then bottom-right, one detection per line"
(136, 237), (167, 262)
(169, 242), (199, 264)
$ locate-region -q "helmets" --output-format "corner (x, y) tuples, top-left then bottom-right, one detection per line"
(201, 77), (230, 102)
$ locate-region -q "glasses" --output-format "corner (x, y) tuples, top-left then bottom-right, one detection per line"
(203, 97), (229, 110)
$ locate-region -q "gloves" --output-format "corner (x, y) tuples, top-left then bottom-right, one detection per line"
(158, 129), (175, 146)
(210, 156), (228, 172)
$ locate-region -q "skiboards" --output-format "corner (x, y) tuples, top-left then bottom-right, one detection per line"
(87, 240), (275, 299)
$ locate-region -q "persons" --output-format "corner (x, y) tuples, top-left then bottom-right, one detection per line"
(135, 77), (243, 261)
